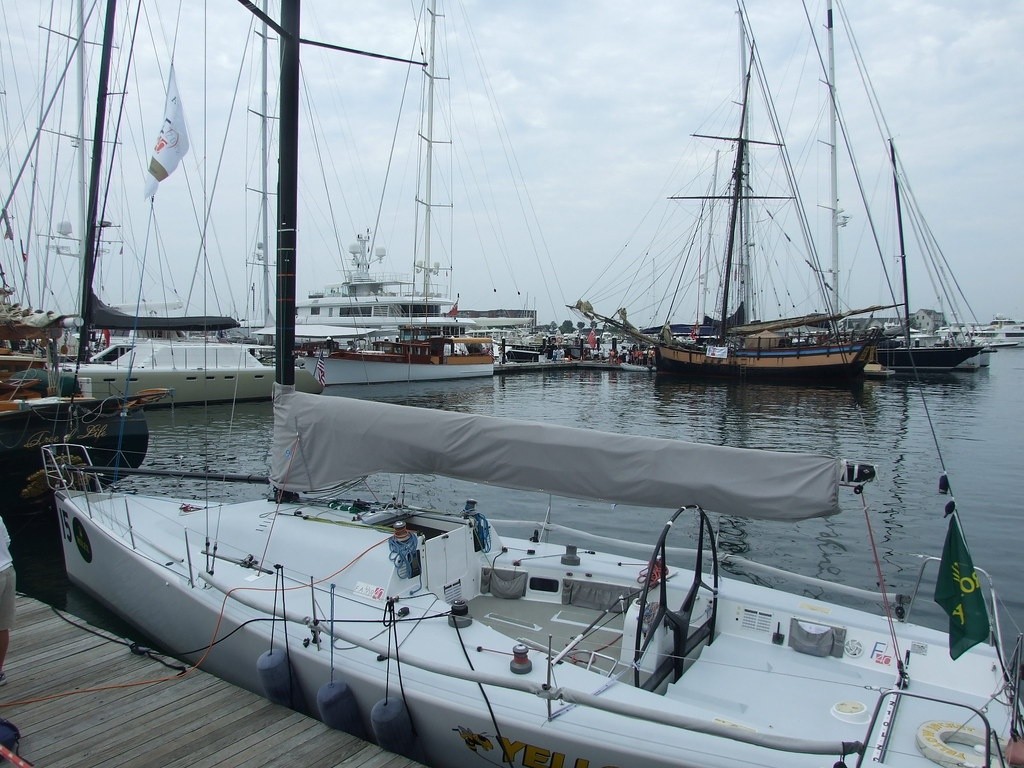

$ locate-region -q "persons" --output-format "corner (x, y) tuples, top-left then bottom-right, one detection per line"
(552, 348), (559, 363)
(560, 347), (565, 363)
(582, 347), (605, 363)
(1, 515), (16, 689)
(608, 345), (656, 365)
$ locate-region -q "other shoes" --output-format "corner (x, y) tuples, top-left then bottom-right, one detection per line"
(0, 672), (7, 685)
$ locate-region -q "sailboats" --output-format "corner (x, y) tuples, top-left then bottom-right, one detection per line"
(39, 0), (1024, 768)
(561, 1), (1024, 379)
(0, 0), (496, 567)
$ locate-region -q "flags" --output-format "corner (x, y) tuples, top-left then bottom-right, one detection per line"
(139, 65), (189, 200)
(587, 325), (596, 349)
(318, 352), (326, 386)
(447, 298), (458, 316)
(934, 512), (991, 661)
